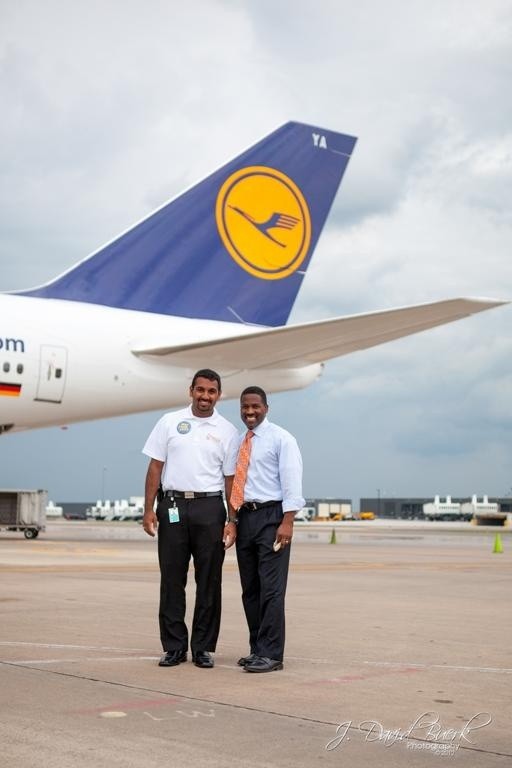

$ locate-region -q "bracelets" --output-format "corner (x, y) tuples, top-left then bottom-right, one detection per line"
(227, 516), (239, 524)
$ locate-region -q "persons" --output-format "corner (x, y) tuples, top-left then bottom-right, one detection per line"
(222, 386), (306, 673)
(140, 369), (240, 667)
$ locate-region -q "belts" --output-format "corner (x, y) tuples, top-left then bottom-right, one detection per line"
(240, 499), (281, 511)
(161, 489), (225, 500)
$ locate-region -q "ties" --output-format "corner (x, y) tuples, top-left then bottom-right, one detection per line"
(228, 431), (256, 510)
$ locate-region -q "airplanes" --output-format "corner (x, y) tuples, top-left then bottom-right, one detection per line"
(0, 119), (512, 440)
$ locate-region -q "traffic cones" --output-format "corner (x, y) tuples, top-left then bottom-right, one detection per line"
(329, 529), (338, 545)
(490, 532), (505, 554)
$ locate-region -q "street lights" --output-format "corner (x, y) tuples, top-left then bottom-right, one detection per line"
(101, 465), (108, 501)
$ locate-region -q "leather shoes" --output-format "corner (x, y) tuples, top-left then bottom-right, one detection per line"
(237, 652), (286, 672)
(191, 647), (216, 669)
(158, 647), (189, 666)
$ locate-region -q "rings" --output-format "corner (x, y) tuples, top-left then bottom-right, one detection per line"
(286, 540), (288, 543)
(143, 527), (146, 529)
